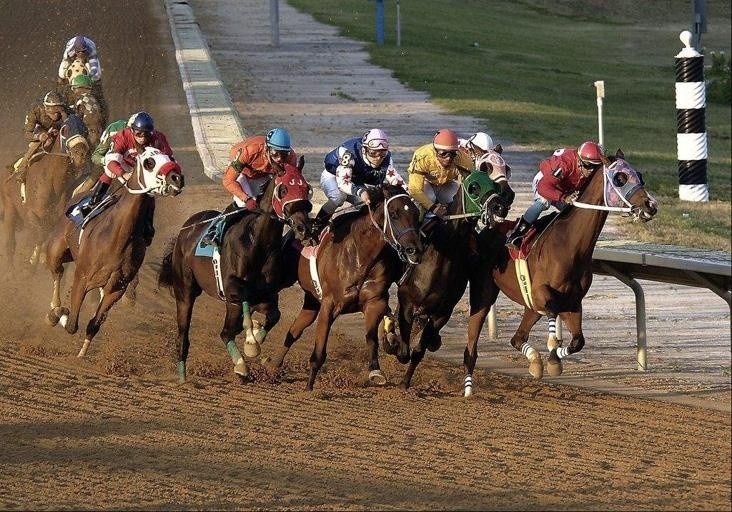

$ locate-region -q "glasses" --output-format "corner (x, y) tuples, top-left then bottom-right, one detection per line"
(435, 149), (458, 160)
(470, 144), (485, 159)
(365, 148), (387, 159)
(580, 159), (600, 171)
(135, 132), (154, 138)
(270, 150), (291, 159)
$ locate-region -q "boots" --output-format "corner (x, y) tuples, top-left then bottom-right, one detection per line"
(18, 142), (51, 183)
(301, 207), (332, 247)
(88, 181), (111, 211)
(505, 215), (532, 250)
(202, 201), (242, 247)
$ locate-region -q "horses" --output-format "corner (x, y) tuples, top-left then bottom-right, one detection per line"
(464, 146), (656, 397)
(274, 181), (422, 391)
(393, 164), (508, 390)
(393, 140), (516, 346)
(60, 54), (94, 89)
(45, 138), (184, 360)
(72, 92), (104, 144)
(156, 147), (312, 385)
(0, 104), (91, 274)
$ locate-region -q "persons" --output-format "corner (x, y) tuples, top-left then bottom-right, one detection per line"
(407, 127), (476, 225)
(456, 130), (494, 167)
(87, 109), (177, 239)
(299, 126), (409, 251)
(57, 32), (102, 96)
(200, 125), (298, 251)
(75, 118), (129, 196)
(504, 140), (605, 253)
(14, 88), (73, 186)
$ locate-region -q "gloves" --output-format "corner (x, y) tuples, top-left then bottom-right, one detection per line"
(245, 198), (259, 212)
(428, 202), (448, 221)
(564, 193), (578, 206)
(38, 132), (49, 144)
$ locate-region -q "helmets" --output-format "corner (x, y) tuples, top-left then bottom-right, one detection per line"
(433, 128), (460, 152)
(75, 38), (88, 52)
(468, 132), (495, 153)
(577, 141), (602, 167)
(361, 128), (389, 151)
(70, 75), (94, 90)
(43, 89), (65, 106)
(266, 128), (292, 152)
(131, 111), (154, 132)
